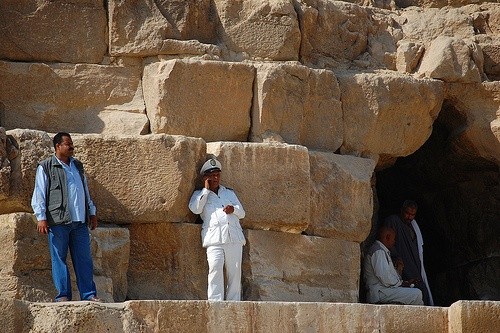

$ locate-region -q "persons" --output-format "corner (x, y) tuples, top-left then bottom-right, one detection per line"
(31, 132), (107, 303)
(362, 199), (434, 306)
(188, 158), (246, 301)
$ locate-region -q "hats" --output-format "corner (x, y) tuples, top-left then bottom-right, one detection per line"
(200, 158), (222, 174)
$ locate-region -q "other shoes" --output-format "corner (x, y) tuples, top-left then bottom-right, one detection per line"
(59, 296), (70, 301)
(89, 296), (105, 302)
